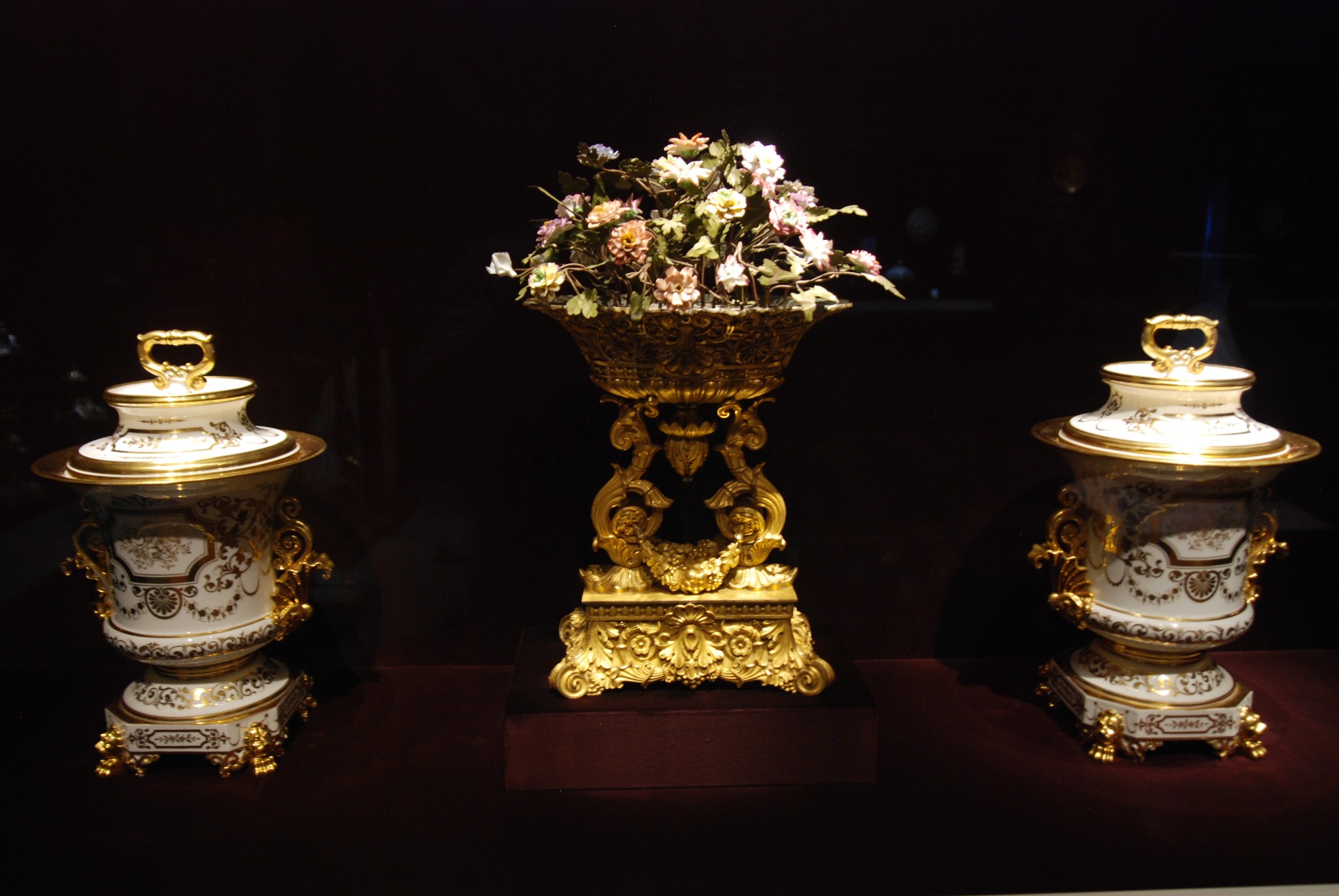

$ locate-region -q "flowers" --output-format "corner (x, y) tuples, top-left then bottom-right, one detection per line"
(485, 130), (907, 321)
